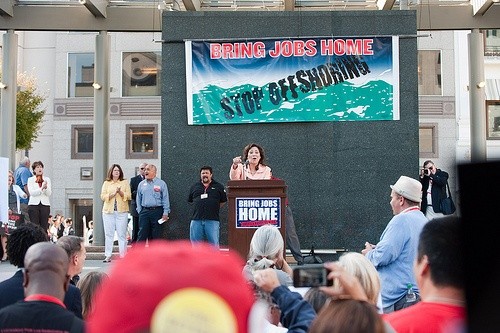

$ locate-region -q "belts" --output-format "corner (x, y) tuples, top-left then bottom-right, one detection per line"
(143, 207), (162, 210)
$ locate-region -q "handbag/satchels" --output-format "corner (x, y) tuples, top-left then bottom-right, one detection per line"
(440, 194), (456, 215)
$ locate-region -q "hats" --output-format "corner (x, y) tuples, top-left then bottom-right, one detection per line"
(389, 175), (423, 204)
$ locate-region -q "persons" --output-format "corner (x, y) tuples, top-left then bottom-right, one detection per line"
(229, 143), (273, 180)
(0, 170), (28, 262)
(27, 160), (52, 238)
(46, 213), (75, 244)
(0, 223), (399, 333)
(381, 214), (467, 333)
(136, 163), (171, 249)
(99, 163), (132, 263)
(268, 176), (305, 266)
(129, 162), (148, 249)
(418, 159), (450, 222)
(361, 175), (430, 314)
(187, 165), (227, 251)
(14, 156), (34, 224)
(86, 212), (133, 246)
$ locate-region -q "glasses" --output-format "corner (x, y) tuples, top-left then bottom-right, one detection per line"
(429, 165), (434, 170)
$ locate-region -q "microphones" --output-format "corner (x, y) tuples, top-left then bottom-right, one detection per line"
(243, 160), (249, 164)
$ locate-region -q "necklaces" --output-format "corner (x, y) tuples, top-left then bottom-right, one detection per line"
(243, 164), (267, 174)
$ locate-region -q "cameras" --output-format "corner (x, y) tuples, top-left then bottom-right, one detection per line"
(293, 264), (334, 287)
(423, 169), (432, 174)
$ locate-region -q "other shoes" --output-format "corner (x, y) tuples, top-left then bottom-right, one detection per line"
(103, 258), (112, 262)
